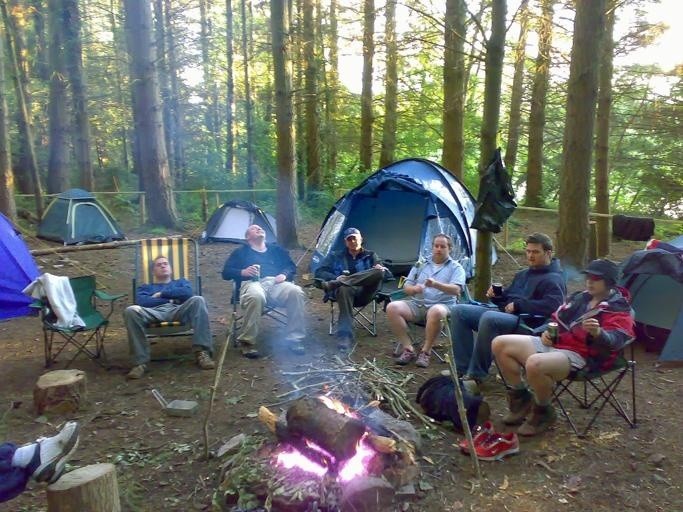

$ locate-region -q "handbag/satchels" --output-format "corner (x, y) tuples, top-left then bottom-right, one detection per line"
(415, 374), (492, 437)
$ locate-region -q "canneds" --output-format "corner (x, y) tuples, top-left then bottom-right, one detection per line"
(343, 270), (350, 276)
(548, 323), (559, 344)
(252, 265), (260, 281)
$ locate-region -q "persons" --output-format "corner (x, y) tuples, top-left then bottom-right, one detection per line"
(221, 224), (306, 358)
(122, 256), (216, 379)
(491, 258), (635, 438)
(0, 422), (80, 503)
(385, 234), (466, 368)
(450, 231), (567, 396)
(314, 228), (385, 349)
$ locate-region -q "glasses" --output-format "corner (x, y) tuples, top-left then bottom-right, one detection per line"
(584, 273), (604, 281)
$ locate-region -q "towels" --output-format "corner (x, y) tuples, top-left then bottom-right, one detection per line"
(22, 273), (85, 329)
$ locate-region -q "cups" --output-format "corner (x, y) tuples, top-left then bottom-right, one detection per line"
(490, 274), (503, 296)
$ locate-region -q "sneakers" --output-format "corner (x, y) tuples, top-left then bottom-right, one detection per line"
(195, 350), (216, 370)
(321, 279), (341, 294)
(126, 361), (151, 380)
(288, 341), (305, 355)
(25, 419), (82, 486)
(238, 341), (260, 359)
(472, 429), (520, 464)
(457, 419), (494, 454)
(414, 349), (432, 368)
(394, 344), (415, 367)
(335, 335), (352, 352)
(438, 368), (482, 397)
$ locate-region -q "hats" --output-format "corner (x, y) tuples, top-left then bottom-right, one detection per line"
(578, 258), (619, 277)
(341, 226), (363, 241)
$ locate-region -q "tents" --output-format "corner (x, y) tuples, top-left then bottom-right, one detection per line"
(36, 187), (126, 246)
(0, 212), (42, 321)
(196, 200), (277, 245)
(616, 236), (683, 363)
(305, 157), (498, 285)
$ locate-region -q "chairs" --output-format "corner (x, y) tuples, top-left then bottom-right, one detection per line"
(495, 268), (567, 388)
(552, 308), (636, 439)
(387, 254), (478, 363)
(231, 273), (293, 346)
(324, 273), (384, 337)
(131, 234), (201, 365)
(41, 274), (128, 371)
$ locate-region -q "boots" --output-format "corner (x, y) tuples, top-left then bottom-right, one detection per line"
(516, 400), (558, 437)
(500, 383), (535, 427)
